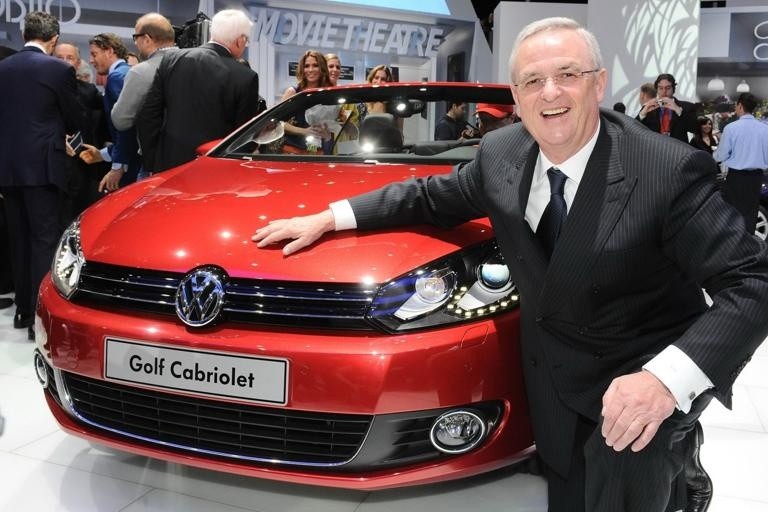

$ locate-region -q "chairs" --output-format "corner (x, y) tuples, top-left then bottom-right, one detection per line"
(348, 113), (410, 157)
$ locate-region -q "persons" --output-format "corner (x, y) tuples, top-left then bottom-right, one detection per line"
(688, 116), (718, 155)
(432, 96), (467, 139)
(456, 102), (523, 147)
(635, 73), (698, 145)
(322, 54), (360, 155)
(2, 7), (264, 343)
(710, 91), (768, 235)
(357, 65), (405, 143)
(633, 81), (657, 122)
(247, 16), (768, 510)
(613, 102), (626, 114)
(277, 49), (332, 156)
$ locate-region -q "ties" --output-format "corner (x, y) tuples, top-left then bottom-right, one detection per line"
(533, 167), (569, 263)
(662, 108), (670, 132)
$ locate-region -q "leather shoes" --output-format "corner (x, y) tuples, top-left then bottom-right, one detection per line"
(0, 286), (12, 295)
(0, 298), (14, 309)
(14, 312), (35, 329)
(683, 421), (714, 511)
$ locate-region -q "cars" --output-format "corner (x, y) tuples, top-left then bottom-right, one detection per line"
(709, 171), (768, 245)
(22, 79), (543, 501)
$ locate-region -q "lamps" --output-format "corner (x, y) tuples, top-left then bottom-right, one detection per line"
(737, 77), (750, 93)
(708, 74), (725, 91)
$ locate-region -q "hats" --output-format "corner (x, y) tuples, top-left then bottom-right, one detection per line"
(472, 102), (515, 121)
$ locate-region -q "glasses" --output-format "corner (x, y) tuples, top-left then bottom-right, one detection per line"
(513, 68), (601, 93)
(133, 32), (153, 41)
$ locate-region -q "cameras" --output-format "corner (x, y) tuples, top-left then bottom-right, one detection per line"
(656, 100), (669, 107)
